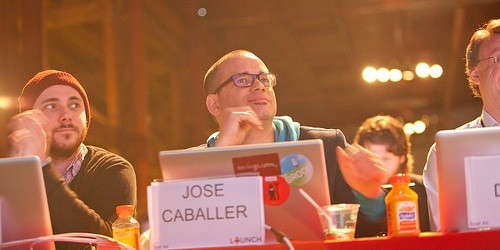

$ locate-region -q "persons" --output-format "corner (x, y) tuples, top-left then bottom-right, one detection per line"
(8, 70), (138, 248)
(354, 115), (431, 233)
(188, 50), (388, 238)
(422, 19), (500, 232)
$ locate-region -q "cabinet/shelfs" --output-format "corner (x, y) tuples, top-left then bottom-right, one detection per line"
(39, 0), (185, 212)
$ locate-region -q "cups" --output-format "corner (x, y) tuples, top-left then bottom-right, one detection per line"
(317, 204), (361, 241)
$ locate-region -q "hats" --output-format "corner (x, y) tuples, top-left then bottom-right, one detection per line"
(17, 69), (91, 129)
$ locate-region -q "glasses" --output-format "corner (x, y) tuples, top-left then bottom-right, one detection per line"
(211, 71), (277, 94)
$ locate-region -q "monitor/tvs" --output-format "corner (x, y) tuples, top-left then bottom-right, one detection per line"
(0, 156), (55, 250)
(436, 127), (500, 234)
(158, 138), (331, 244)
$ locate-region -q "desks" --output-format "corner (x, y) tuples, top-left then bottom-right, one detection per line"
(192, 227), (500, 250)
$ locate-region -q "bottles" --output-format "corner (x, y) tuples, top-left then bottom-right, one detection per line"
(384, 173), (420, 236)
(112, 205), (140, 250)
